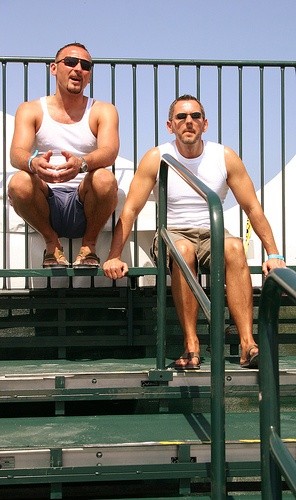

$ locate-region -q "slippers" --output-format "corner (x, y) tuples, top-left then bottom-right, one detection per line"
(240, 344), (259, 367)
(72, 253), (100, 269)
(174, 351), (201, 369)
(42, 246), (69, 266)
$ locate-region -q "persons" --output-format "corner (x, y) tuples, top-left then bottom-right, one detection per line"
(7, 43), (120, 270)
(103, 94), (288, 369)
(267, 254), (285, 260)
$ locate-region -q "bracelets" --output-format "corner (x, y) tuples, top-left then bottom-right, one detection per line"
(28, 149), (39, 173)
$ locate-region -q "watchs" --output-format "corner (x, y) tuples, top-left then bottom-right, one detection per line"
(79, 157), (89, 175)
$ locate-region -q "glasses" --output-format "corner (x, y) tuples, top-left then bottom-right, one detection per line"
(56, 57), (94, 71)
(175, 112), (201, 119)
